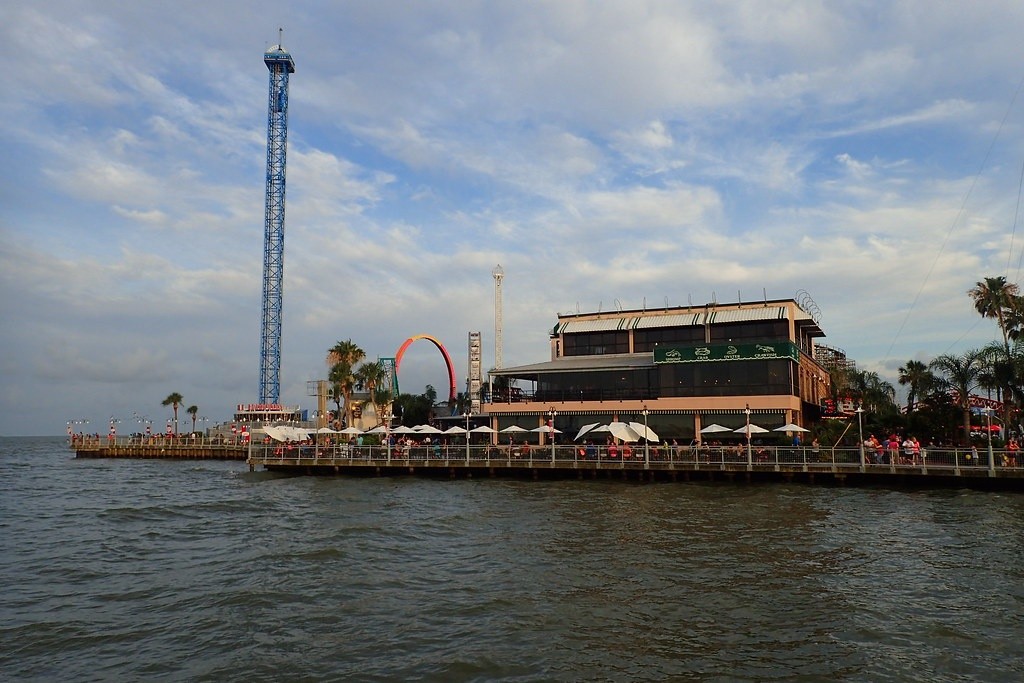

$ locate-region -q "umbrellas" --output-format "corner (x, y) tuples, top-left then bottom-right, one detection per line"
(573, 422), (659, 442)
(732, 423), (769, 443)
(959, 424), (1000, 434)
(773, 423), (812, 438)
(699, 423), (733, 442)
(260, 424), (563, 446)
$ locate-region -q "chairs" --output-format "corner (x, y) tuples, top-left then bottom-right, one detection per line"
(366, 448), (502, 459)
(513, 448), (774, 462)
(274, 448), (330, 459)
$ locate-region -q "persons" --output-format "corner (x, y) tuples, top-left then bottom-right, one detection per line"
(72, 432), (244, 447)
(263, 434), (573, 459)
(663, 438), (764, 465)
(582, 435), (633, 461)
(855, 431), (1024, 471)
(792, 435), (802, 463)
(809, 436), (821, 463)
